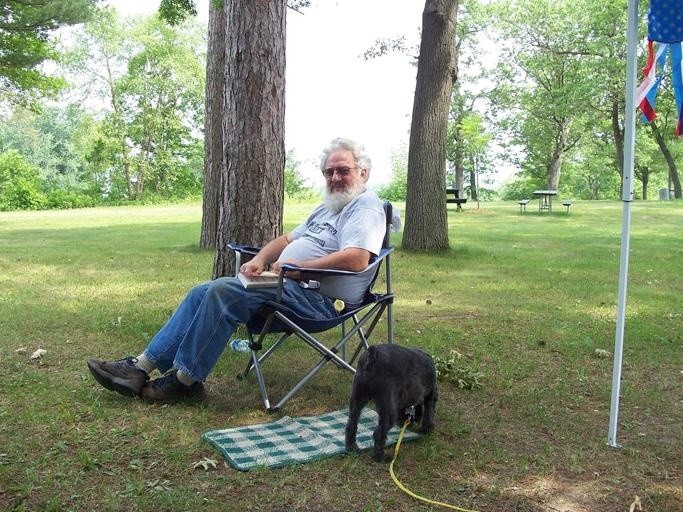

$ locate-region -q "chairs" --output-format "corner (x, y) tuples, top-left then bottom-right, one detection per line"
(225, 200), (396, 413)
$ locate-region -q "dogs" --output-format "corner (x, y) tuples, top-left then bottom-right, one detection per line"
(344, 343), (439, 464)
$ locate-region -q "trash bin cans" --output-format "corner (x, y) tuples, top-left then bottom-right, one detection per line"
(669, 190), (676, 201)
(659, 187), (669, 201)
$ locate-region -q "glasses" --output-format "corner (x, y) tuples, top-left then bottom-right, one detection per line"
(322, 166), (359, 177)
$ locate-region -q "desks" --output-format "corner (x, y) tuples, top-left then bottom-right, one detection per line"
(533, 189), (557, 214)
(445, 189), (461, 209)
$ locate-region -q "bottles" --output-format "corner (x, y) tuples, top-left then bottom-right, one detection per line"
(230, 339), (252, 352)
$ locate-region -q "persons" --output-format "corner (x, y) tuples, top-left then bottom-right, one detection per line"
(87, 137), (386, 402)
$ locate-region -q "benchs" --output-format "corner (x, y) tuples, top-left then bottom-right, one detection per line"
(563, 199), (571, 215)
(447, 198), (466, 203)
(518, 199), (529, 214)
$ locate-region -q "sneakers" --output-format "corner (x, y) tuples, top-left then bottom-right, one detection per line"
(87, 356), (207, 405)
